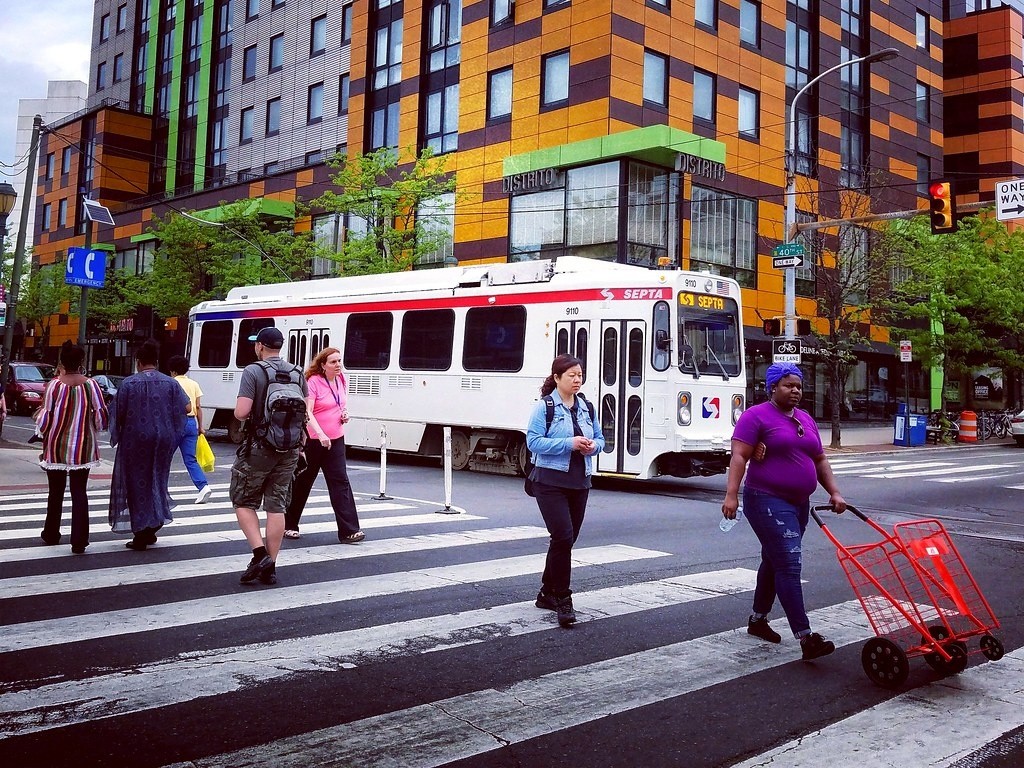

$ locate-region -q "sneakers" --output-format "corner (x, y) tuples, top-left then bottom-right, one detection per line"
(284, 528), (299, 539)
(747, 613), (780, 645)
(71, 545), (87, 554)
(341, 531), (365, 545)
(195, 485), (212, 505)
(126, 541), (147, 551)
(41, 532), (59, 545)
(800, 631), (835, 660)
(557, 598), (576, 624)
(240, 556), (273, 580)
(535, 590), (561, 613)
(256, 565), (277, 584)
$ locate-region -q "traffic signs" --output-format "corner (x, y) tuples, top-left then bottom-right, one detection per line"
(899, 340), (912, 362)
(772, 243), (804, 257)
(994, 178), (1024, 221)
(772, 256), (804, 268)
(772, 339), (801, 366)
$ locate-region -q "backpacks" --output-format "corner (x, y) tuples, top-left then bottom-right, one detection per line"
(524, 396), (557, 499)
(253, 361), (307, 452)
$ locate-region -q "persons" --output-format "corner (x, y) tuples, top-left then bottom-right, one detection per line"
(0, 384), (8, 422)
(719, 363), (849, 661)
(228, 324), (309, 589)
(33, 339), (108, 555)
(280, 348), (367, 546)
(524, 354), (606, 623)
(165, 355), (213, 505)
(106, 340), (194, 551)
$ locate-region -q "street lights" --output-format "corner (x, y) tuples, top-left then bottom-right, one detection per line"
(0, 179), (19, 279)
(785, 46), (898, 369)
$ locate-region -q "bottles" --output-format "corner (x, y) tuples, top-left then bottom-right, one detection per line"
(719, 507), (744, 532)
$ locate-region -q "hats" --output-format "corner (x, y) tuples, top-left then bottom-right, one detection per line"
(247, 328), (284, 348)
(766, 362), (804, 390)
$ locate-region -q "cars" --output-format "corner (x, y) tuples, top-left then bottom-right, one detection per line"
(746, 376), (897, 421)
(89, 375), (126, 406)
(1010, 410), (1024, 447)
(0, 362), (59, 416)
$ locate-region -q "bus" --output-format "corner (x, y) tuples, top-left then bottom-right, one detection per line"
(182, 256), (748, 484)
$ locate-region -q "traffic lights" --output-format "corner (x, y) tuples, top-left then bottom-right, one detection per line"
(763, 319), (781, 335)
(927, 176), (958, 235)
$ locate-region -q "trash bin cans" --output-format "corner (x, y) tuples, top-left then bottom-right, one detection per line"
(892, 403), (928, 447)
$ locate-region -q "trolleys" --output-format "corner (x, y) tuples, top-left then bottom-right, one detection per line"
(810, 502), (1005, 689)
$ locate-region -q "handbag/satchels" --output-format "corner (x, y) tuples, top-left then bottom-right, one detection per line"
(195, 434), (217, 474)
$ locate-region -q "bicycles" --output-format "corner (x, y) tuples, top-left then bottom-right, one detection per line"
(911, 408), (1023, 443)
